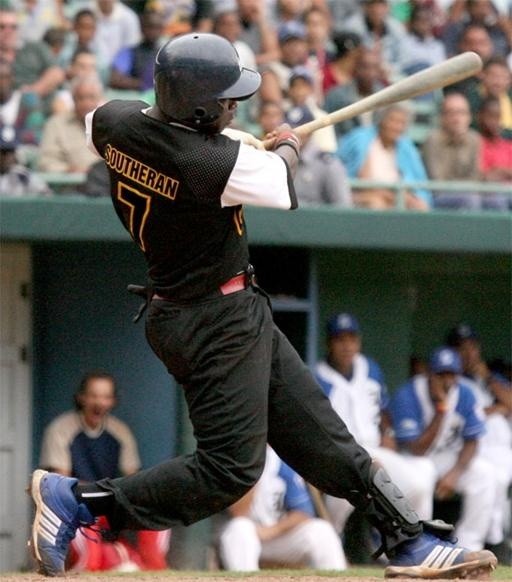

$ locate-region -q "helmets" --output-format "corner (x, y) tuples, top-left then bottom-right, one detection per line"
(153, 31), (263, 123)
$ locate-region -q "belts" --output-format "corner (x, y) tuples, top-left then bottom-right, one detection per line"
(152, 271), (257, 299)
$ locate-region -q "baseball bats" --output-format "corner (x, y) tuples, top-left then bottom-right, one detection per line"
(259, 50), (483, 151)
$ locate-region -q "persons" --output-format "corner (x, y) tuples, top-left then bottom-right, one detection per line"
(38, 374), (169, 568)
(30, 32), (498, 582)
(214, 313), (510, 570)
(0, 1), (512, 212)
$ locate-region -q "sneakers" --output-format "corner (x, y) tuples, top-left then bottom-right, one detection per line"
(385, 535), (497, 576)
(30, 470), (95, 576)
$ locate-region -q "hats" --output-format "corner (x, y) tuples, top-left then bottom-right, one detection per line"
(278, 21), (306, 43)
(432, 347), (463, 375)
(289, 68), (313, 85)
(328, 312), (358, 339)
